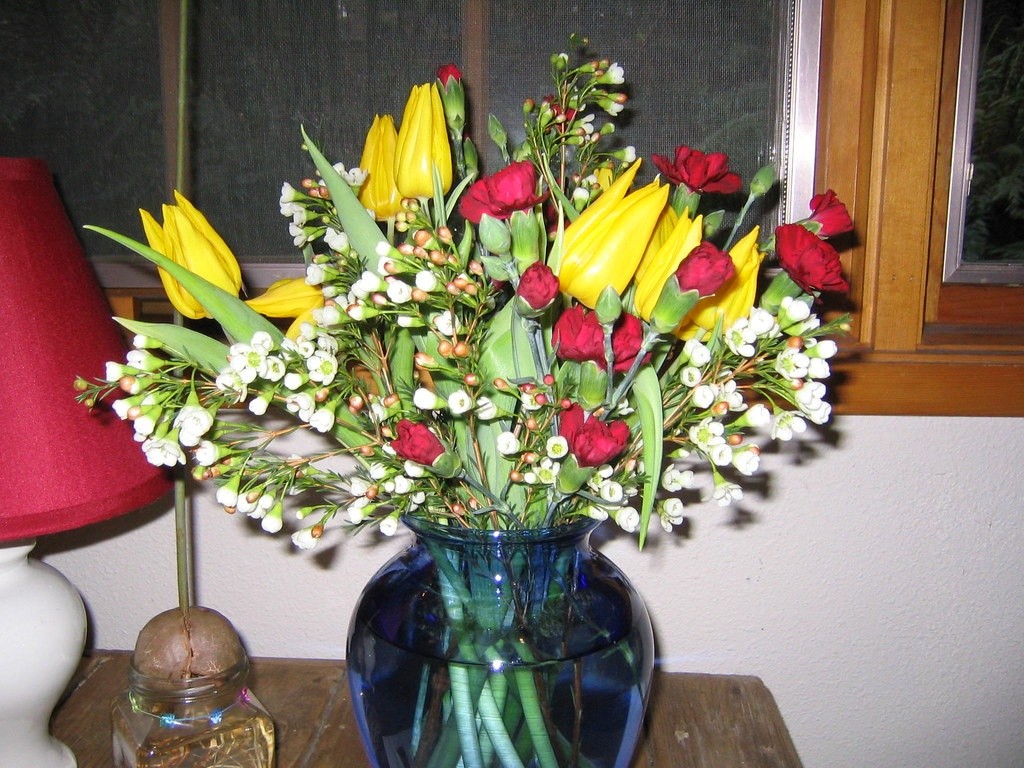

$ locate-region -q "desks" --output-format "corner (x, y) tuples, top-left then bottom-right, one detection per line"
(48, 647), (807, 768)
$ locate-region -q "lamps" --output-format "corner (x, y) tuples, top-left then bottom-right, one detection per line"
(0, 154), (176, 767)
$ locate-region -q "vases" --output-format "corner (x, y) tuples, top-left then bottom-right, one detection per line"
(347, 512), (655, 768)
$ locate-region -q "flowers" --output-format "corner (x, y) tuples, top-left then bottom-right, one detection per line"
(70, 31), (854, 767)
